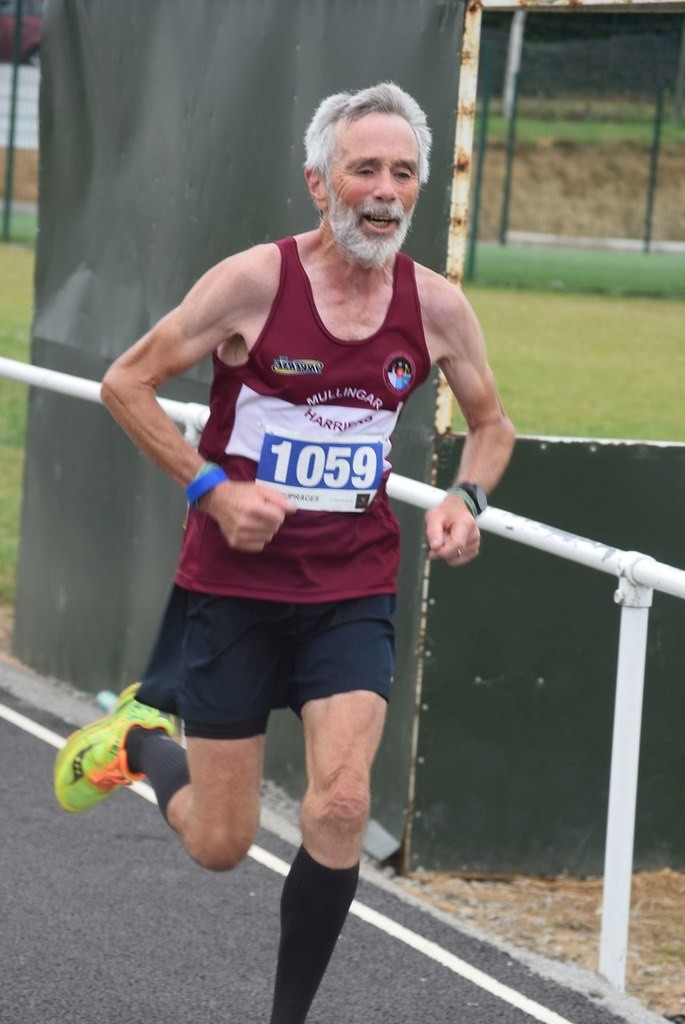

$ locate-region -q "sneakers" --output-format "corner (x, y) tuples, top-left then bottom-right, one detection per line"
(54, 680), (183, 813)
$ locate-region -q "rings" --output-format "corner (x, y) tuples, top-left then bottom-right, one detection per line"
(457, 548), (462, 559)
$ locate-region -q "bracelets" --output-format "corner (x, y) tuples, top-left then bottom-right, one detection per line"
(448, 482), (487, 518)
(184, 461), (228, 509)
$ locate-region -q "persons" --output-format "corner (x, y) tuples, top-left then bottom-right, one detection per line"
(55, 83), (515, 1024)
